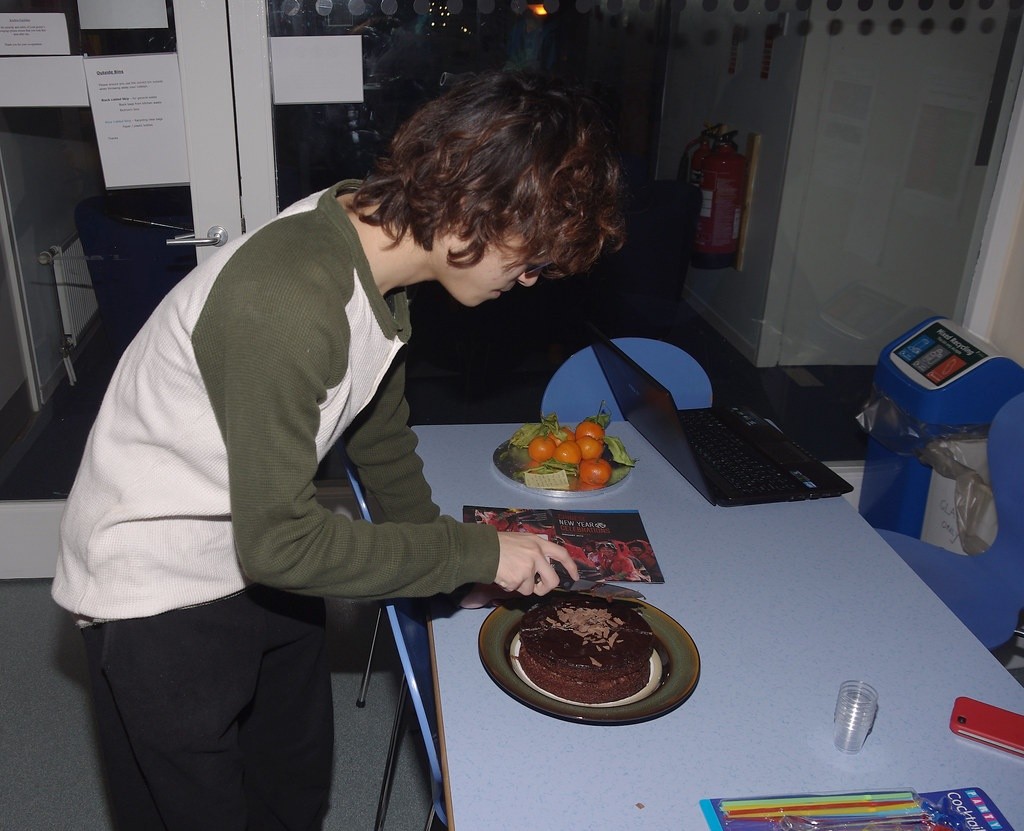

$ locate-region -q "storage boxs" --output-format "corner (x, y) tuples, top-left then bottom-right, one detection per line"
(915, 434), (998, 555)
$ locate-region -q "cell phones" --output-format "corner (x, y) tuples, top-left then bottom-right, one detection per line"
(949, 697), (1024, 760)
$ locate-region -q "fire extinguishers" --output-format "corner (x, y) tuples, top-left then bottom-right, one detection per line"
(680, 122), (743, 255)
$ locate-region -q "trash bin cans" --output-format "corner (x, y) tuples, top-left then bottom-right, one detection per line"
(854, 317), (1024, 537)
(920, 437), (1000, 557)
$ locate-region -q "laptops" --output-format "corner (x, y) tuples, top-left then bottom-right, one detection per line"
(582, 320), (854, 506)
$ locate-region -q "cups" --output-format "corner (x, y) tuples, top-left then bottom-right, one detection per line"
(832, 680), (878, 755)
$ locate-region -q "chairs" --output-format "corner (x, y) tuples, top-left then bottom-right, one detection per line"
(337, 436), (450, 830)
(540, 336), (715, 423)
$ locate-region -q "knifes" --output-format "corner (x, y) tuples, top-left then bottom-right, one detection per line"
(535, 572), (644, 599)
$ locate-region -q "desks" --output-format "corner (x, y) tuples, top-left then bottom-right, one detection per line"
(406, 423), (1023, 831)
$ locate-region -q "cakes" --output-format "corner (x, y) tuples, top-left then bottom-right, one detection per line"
(517, 599), (657, 703)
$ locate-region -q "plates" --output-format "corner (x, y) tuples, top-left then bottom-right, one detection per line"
(493, 439), (630, 499)
(477, 598), (701, 723)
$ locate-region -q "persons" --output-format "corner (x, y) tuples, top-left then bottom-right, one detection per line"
(51, 68), (630, 830)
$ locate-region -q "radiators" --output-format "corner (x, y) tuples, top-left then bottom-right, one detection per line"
(38, 230), (100, 353)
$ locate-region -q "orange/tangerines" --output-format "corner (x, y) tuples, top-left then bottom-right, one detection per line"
(529, 421), (610, 486)
